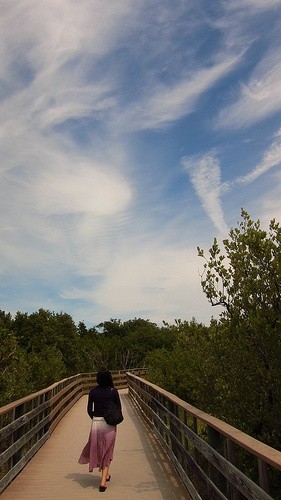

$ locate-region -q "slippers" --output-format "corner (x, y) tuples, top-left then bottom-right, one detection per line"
(100, 486), (106, 492)
(106, 475), (111, 481)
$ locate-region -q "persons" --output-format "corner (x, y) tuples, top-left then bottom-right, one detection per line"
(78, 369), (124, 492)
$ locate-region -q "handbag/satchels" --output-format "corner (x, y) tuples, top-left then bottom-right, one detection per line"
(104, 387), (124, 425)
